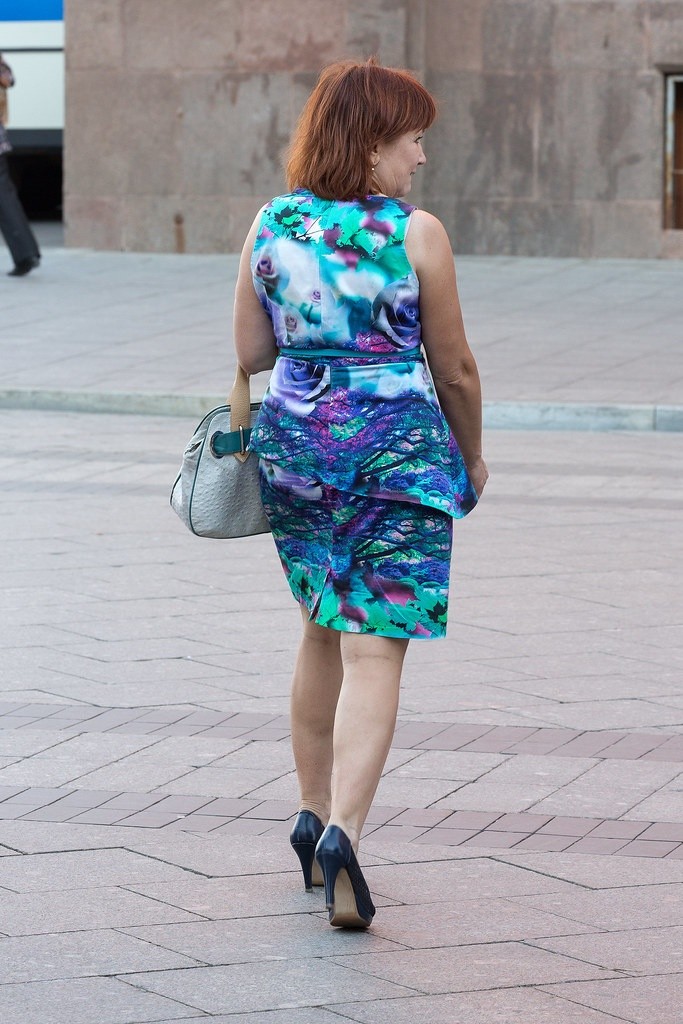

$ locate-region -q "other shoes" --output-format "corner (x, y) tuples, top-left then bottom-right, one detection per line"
(6, 258), (40, 276)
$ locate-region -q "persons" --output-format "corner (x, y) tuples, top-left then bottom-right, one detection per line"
(0, 53), (45, 273)
(233, 61), (490, 932)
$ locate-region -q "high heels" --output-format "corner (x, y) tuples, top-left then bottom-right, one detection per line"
(289, 809), (325, 893)
(313, 824), (376, 927)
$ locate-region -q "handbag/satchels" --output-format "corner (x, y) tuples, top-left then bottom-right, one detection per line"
(168, 363), (273, 540)
